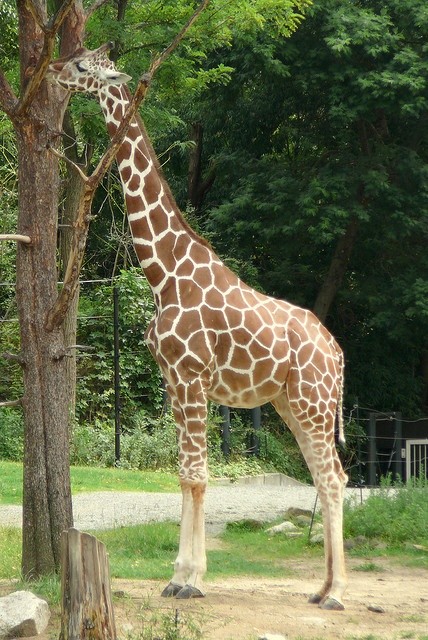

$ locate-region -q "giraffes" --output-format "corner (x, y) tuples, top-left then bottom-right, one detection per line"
(27, 39), (356, 613)
